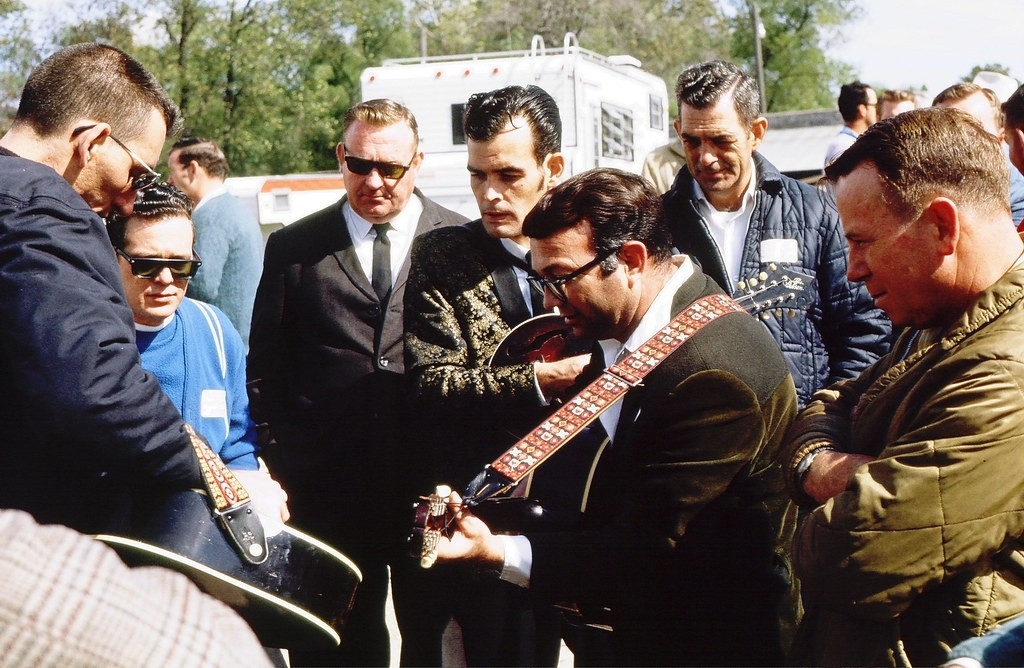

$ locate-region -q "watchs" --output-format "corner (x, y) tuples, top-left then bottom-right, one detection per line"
(796, 447), (837, 482)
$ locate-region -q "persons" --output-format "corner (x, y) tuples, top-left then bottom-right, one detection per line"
(933, 70), (1024, 241)
(169, 131), (266, 355)
(0, 40), (290, 668)
(402, 87), (627, 668)
(777, 106), (1024, 668)
(655, 55), (895, 411)
(245, 96), (471, 668)
(817, 80), (879, 174)
(877, 89), (919, 122)
(104, 170), (265, 477)
(642, 135), (690, 198)
(420, 162), (897, 668)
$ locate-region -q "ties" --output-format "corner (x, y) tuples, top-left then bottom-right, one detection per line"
(372, 223), (396, 305)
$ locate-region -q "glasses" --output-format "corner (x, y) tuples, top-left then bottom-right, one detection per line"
(525, 246), (622, 302)
(344, 142), (417, 179)
(866, 103), (880, 108)
(73, 125), (162, 192)
(115, 247), (203, 279)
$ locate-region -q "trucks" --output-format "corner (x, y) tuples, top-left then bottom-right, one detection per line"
(359, 31), (674, 221)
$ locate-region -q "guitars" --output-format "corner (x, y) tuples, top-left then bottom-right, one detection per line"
(486, 263), (817, 444)
(82, 485), (365, 652)
(406, 406), (644, 633)
